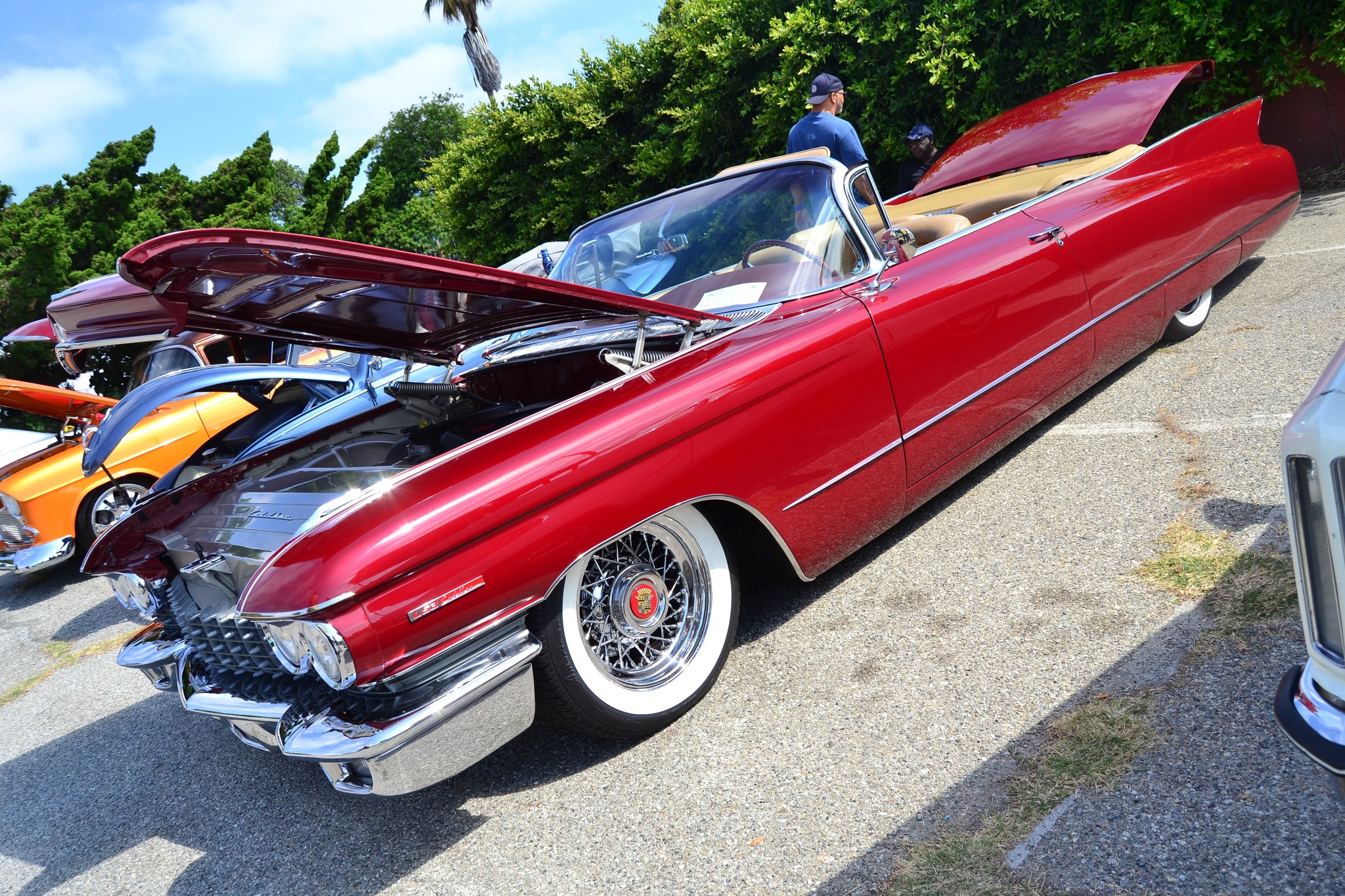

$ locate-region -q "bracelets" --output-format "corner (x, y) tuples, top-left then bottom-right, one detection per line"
(794, 203), (805, 210)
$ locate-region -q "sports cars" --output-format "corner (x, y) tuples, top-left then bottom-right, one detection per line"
(78, 56), (1306, 799)
(0, 234), (568, 576)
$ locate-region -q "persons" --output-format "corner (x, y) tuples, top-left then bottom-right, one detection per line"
(784, 73), (876, 231)
(901, 126), (944, 188)
(359, 285), (458, 355)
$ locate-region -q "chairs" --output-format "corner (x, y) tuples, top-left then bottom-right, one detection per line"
(733, 188), (1046, 276)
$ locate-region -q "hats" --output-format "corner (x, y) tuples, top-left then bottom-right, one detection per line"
(805, 73), (843, 105)
(901, 124), (934, 145)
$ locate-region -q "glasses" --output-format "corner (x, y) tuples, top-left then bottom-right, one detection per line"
(908, 136), (928, 146)
(828, 91), (848, 98)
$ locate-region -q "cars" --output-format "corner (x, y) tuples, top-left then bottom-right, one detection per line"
(1269, 343), (1344, 780)
